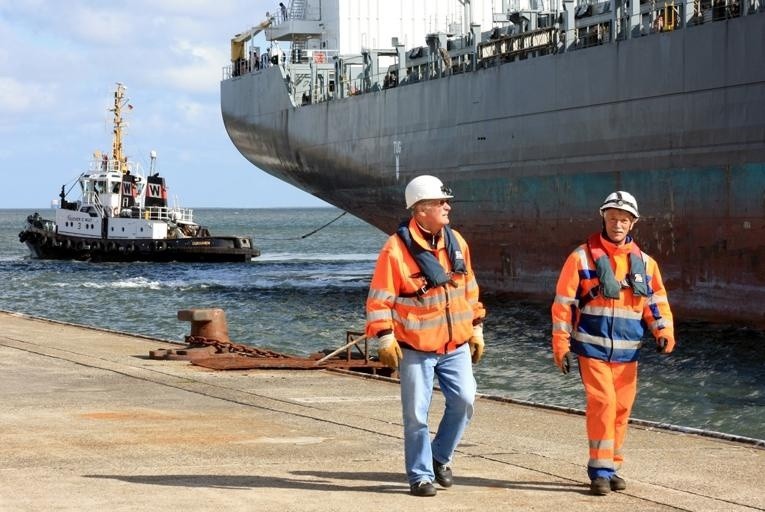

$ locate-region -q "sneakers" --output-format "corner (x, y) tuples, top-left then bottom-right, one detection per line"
(410, 479), (438, 496)
(431, 458), (452, 486)
(592, 472), (626, 495)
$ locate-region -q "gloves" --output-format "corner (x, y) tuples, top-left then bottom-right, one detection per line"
(656, 329), (674, 354)
(377, 332), (403, 369)
(470, 322), (485, 363)
(554, 349), (573, 376)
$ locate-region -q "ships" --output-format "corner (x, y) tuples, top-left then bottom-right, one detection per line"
(217, 0), (765, 331)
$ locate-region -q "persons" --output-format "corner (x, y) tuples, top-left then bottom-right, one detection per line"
(279, 2), (289, 21)
(549, 189), (678, 496)
(365, 174), (489, 498)
(248, 48), (274, 74)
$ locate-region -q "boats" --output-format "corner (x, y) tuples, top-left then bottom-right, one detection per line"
(17, 81), (261, 264)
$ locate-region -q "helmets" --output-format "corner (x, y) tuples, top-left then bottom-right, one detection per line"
(405, 174), (455, 210)
(598, 190), (638, 219)
